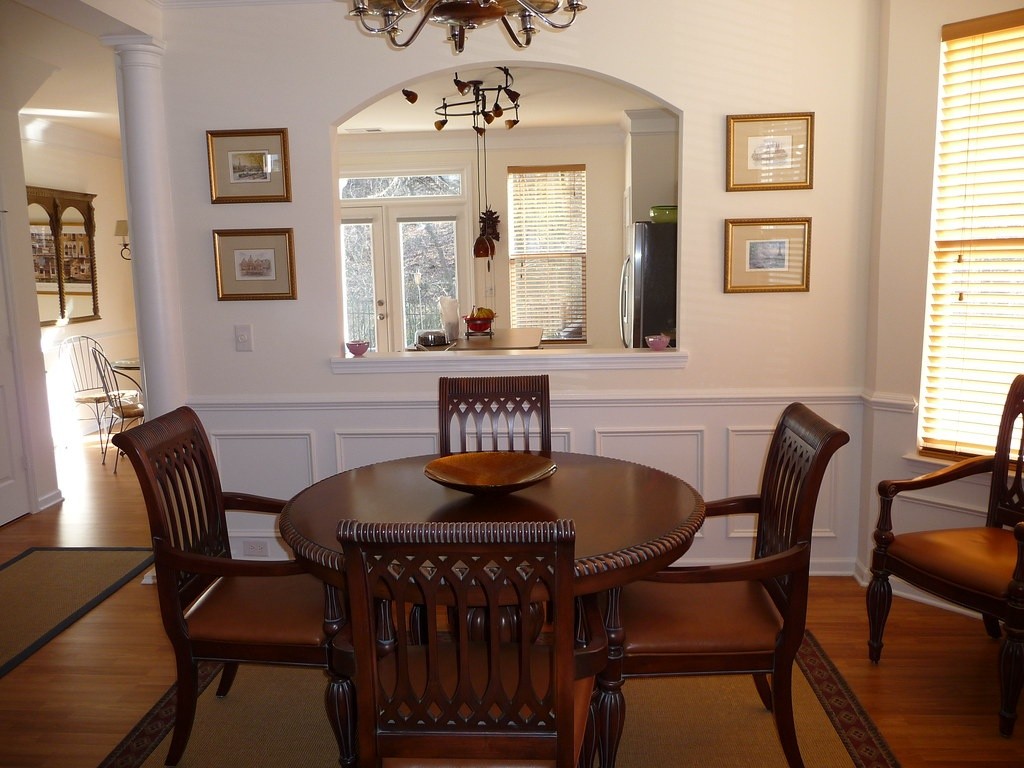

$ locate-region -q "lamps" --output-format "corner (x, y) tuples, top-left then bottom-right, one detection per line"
(114, 219), (131, 260)
(473, 115), (490, 258)
(483, 121), (496, 256)
(347, 0), (588, 54)
(401, 65), (520, 137)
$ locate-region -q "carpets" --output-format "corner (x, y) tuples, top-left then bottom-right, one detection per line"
(96, 628), (902, 768)
(0, 546), (154, 679)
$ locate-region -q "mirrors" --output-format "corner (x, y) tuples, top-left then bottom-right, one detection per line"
(25, 186), (103, 329)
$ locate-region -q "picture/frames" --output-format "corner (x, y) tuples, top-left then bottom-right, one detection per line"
(726, 111), (816, 192)
(29, 221), (59, 296)
(61, 222), (92, 296)
(207, 128), (293, 204)
(725, 217), (813, 294)
(212, 227), (298, 302)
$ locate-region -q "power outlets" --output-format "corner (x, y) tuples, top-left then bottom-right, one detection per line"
(243, 538), (269, 557)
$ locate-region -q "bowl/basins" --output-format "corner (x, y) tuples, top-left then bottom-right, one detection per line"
(346, 341), (369, 356)
(645, 335), (670, 351)
(464, 315), (492, 331)
(649, 205), (678, 223)
(424, 451), (557, 499)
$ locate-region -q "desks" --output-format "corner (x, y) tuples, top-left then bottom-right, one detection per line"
(277, 450), (706, 767)
(111, 357), (142, 392)
(405, 327), (544, 352)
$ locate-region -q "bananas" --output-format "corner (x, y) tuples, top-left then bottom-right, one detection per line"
(470, 305), (495, 319)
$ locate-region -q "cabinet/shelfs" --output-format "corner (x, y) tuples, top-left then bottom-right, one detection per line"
(618, 109), (679, 228)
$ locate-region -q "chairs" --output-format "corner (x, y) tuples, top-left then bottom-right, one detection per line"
(335, 518), (596, 768)
(578, 401), (851, 768)
(438, 375), (552, 455)
(90, 346), (146, 475)
(867, 373), (1024, 741)
(111, 404), (348, 768)
(58, 335), (141, 454)
